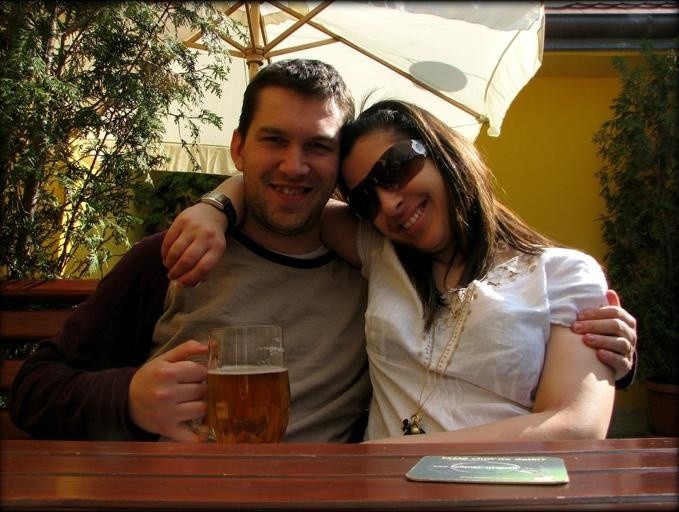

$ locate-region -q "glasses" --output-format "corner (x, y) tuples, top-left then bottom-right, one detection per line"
(346, 138), (427, 221)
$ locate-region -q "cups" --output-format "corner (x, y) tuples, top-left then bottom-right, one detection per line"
(184, 323), (292, 445)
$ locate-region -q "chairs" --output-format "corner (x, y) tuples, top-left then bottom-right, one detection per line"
(0, 279), (101, 440)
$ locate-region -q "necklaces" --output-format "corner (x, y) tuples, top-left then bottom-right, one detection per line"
(400, 284), (474, 435)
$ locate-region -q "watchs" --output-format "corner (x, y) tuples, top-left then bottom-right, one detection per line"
(193, 190), (238, 234)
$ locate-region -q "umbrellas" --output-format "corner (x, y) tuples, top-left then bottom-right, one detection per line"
(42, 1), (547, 180)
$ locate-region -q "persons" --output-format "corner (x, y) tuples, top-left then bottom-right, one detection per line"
(160, 99), (618, 446)
(4, 56), (639, 443)
